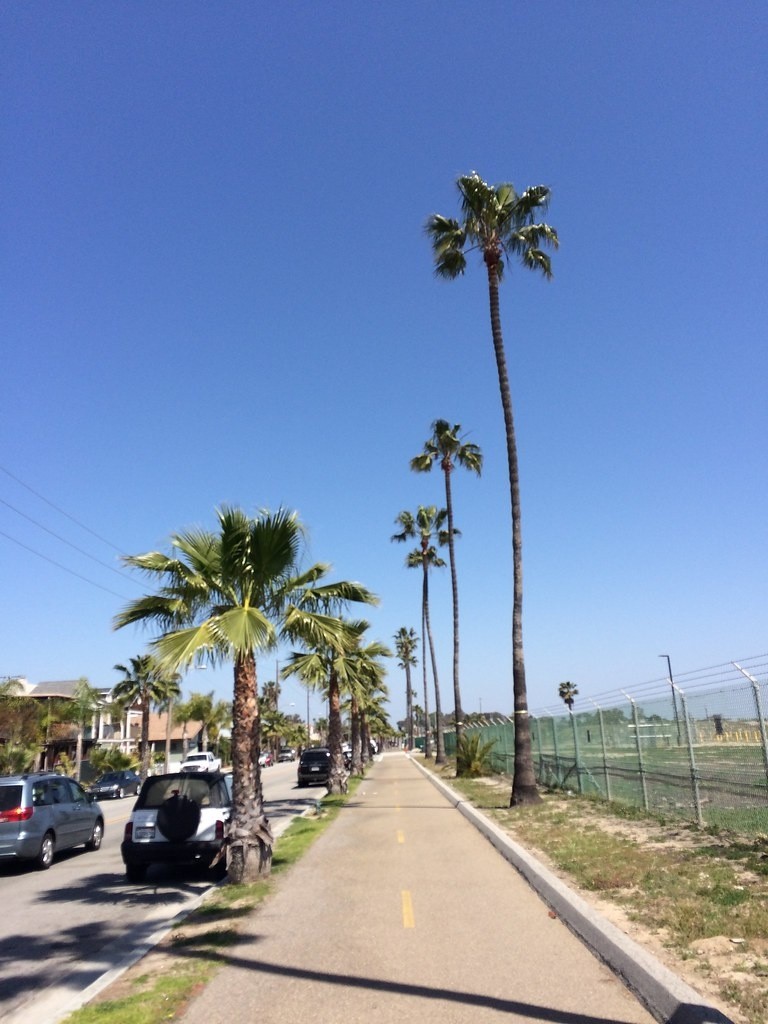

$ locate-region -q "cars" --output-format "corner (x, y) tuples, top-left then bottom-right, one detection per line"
(340, 739), (380, 771)
(86, 771), (142, 801)
(277, 749), (295, 763)
(257, 752), (274, 769)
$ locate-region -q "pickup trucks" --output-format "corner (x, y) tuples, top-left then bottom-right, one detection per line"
(179, 751), (223, 774)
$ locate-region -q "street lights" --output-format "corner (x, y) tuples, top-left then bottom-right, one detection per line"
(165, 665), (209, 774)
(657, 655), (684, 748)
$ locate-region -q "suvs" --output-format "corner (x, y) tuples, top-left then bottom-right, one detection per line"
(120, 771), (235, 882)
(0, 771), (106, 870)
(298, 747), (331, 787)
